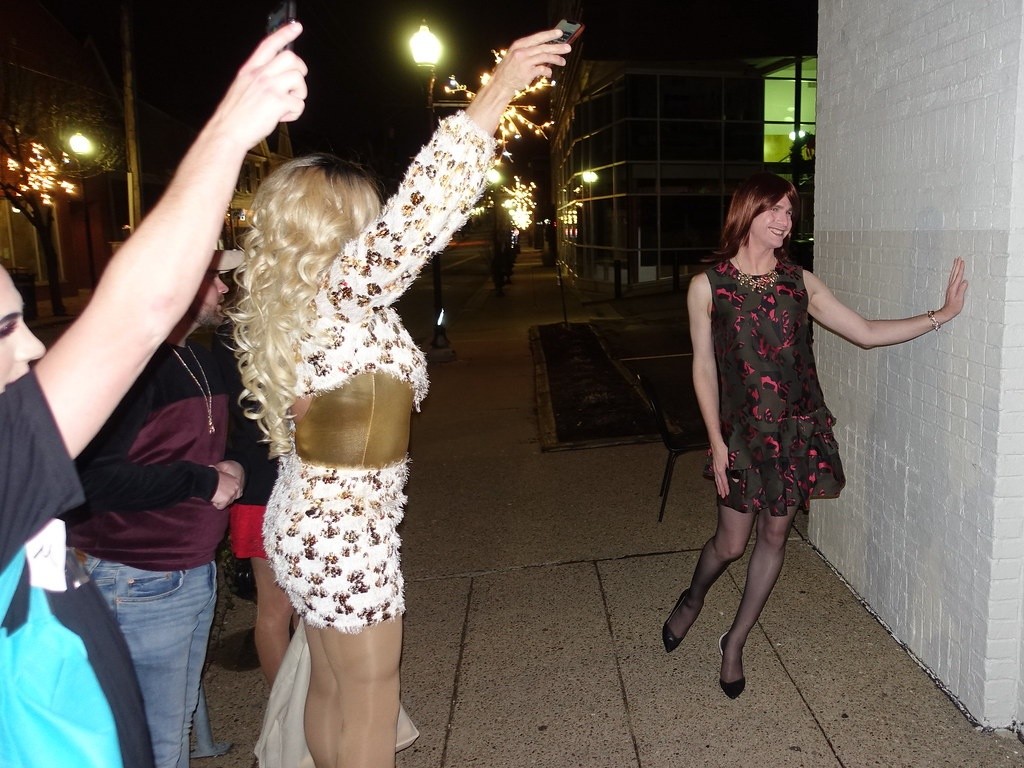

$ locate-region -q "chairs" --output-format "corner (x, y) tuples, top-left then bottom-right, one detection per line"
(635, 369), (730, 522)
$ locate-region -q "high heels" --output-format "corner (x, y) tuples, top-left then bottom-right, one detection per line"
(717, 631), (747, 700)
(663, 588), (705, 654)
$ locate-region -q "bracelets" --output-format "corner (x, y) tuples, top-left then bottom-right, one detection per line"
(927, 309), (942, 333)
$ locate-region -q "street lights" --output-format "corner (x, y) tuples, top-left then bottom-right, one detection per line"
(410, 17), (459, 365)
(486, 170), (504, 301)
(68, 132), (99, 294)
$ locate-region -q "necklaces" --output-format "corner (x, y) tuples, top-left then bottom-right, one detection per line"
(166, 342), (216, 435)
(731, 255), (782, 293)
(227, 30), (574, 768)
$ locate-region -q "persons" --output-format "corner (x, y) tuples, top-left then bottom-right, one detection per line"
(215, 322), (313, 694)
(0, 16), (309, 768)
(660, 171), (969, 701)
(61, 253), (231, 768)
